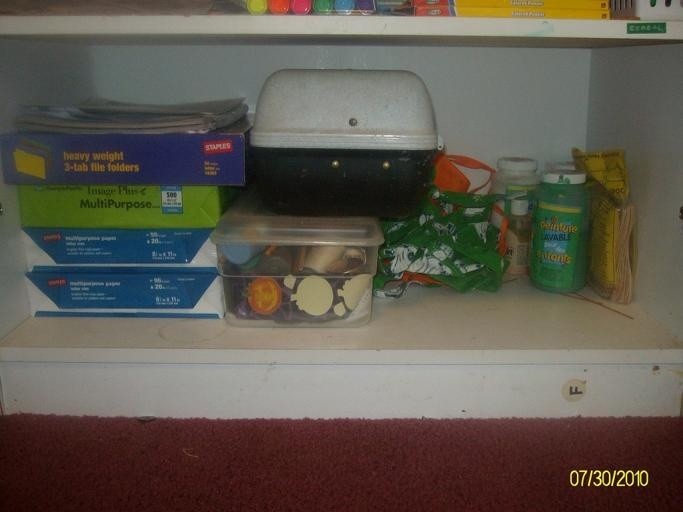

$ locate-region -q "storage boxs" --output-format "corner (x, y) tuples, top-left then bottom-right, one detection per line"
(15, 184), (236, 227)
(19, 227), (218, 265)
(0, 114), (254, 185)
(21, 268), (225, 318)
(209, 183), (385, 327)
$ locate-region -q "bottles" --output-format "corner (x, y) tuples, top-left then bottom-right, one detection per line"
(504, 197), (531, 277)
(530, 171), (592, 294)
(489, 157), (537, 238)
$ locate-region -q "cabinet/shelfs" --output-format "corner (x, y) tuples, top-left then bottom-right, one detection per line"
(0, 13), (683, 421)
